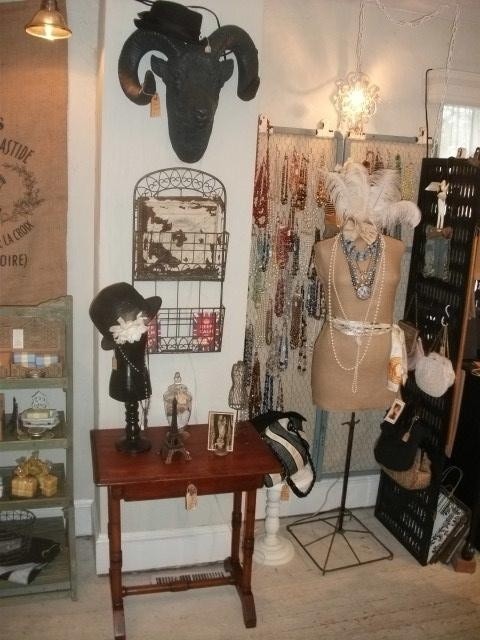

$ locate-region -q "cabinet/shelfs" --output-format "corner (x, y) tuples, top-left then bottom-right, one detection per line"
(1, 295), (80, 601)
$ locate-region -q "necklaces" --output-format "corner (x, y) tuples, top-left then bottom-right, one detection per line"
(245, 141), (406, 425)
(325, 231), (389, 397)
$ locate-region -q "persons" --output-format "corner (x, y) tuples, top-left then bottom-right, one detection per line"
(307, 160), (424, 415)
(89, 282), (163, 405)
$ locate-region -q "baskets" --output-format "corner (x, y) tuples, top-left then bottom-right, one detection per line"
(0, 508), (36, 564)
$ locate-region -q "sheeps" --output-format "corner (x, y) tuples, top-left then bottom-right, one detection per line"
(117, 25), (260, 163)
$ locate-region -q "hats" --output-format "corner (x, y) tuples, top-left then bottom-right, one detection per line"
(90, 281), (162, 349)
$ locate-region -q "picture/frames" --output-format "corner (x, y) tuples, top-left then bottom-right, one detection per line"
(206, 409), (238, 456)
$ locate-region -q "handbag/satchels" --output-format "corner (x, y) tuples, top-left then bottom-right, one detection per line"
(372, 413), (432, 491)
(253, 410), (309, 487)
(397, 319), (457, 398)
(427, 466), (472, 564)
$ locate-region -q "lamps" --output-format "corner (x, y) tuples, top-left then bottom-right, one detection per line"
(333, 1), (464, 157)
(25, 0), (73, 41)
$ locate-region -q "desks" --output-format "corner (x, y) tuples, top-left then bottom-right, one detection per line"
(89, 419), (284, 640)
